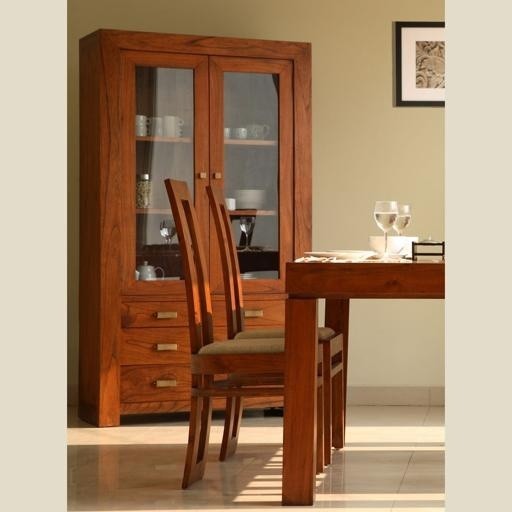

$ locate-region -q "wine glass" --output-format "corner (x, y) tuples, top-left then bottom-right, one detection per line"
(160, 219), (177, 245)
(374, 201), (399, 255)
(239, 216), (256, 251)
(393, 205), (412, 255)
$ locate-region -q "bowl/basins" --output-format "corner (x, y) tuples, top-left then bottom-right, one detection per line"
(237, 189), (266, 208)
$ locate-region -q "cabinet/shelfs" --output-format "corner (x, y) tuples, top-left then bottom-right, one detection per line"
(80, 29), (312, 429)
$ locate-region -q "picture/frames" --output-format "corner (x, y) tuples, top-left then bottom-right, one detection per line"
(392, 22), (445, 106)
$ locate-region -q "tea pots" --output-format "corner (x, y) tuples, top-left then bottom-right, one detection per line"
(136, 260), (165, 279)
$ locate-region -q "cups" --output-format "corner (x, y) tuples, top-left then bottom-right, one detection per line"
(225, 198), (236, 210)
(149, 117), (163, 135)
(136, 115), (148, 136)
(248, 124), (270, 138)
(164, 116), (184, 137)
(234, 127), (248, 138)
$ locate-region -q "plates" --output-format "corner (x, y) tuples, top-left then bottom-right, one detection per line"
(303, 249), (376, 261)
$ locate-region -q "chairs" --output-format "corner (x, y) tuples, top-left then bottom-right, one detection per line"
(164, 179), (323, 489)
(207, 186), (342, 465)
